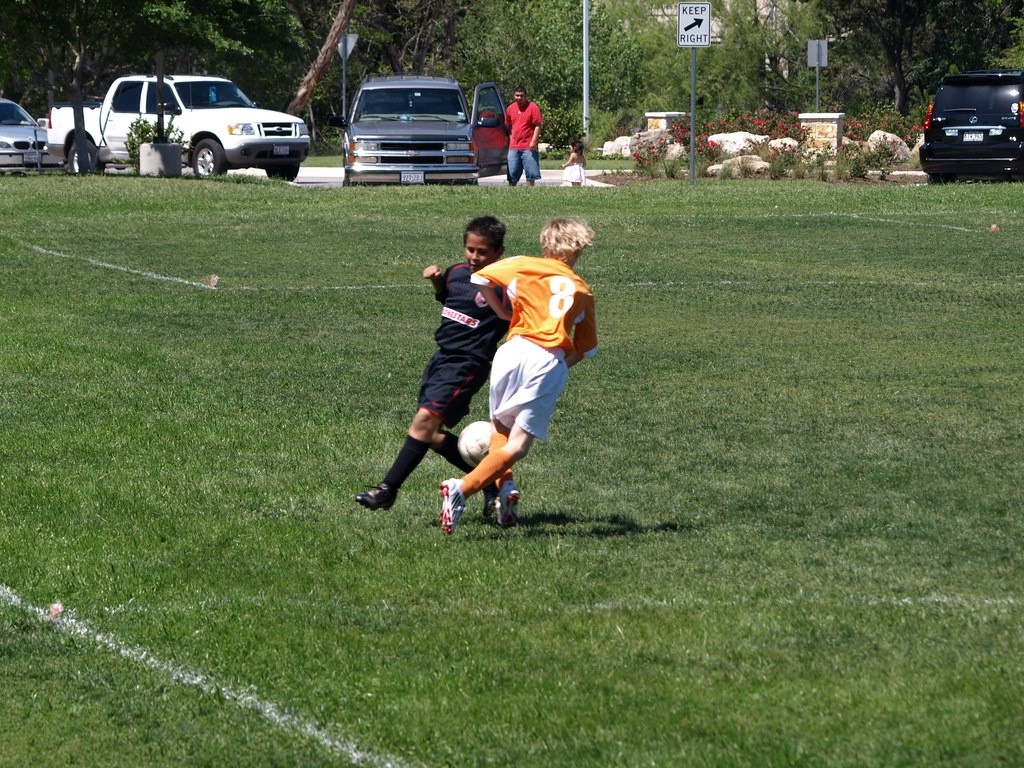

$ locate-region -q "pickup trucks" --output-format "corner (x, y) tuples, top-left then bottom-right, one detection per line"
(43, 71), (312, 183)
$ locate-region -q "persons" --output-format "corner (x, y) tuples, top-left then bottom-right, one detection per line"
(355, 215), (599, 536)
(505, 85), (542, 187)
(562, 141), (586, 188)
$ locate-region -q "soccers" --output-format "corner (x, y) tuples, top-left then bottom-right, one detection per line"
(456, 420), (495, 470)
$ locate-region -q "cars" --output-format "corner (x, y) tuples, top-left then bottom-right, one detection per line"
(0, 99), (51, 174)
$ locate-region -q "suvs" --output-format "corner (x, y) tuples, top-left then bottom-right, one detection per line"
(327, 72), (511, 186)
(918, 67), (1024, 181)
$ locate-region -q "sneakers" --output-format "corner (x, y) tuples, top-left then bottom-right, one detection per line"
(439, 478), (467, 535)
(494, 479), (520, 526)
(356, 482), (397, 510)
(482, 482), (500, 520)
